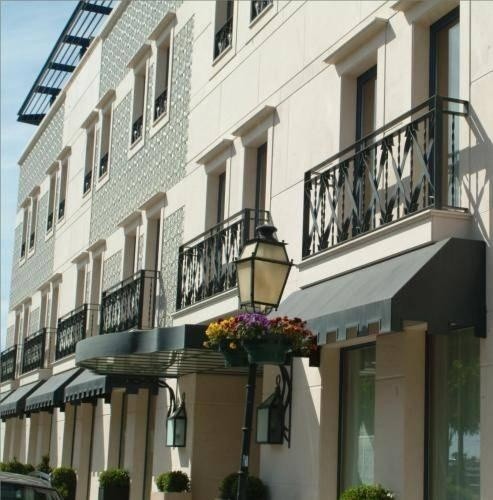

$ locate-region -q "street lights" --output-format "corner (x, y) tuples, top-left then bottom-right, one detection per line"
(232, 222), (294, 500)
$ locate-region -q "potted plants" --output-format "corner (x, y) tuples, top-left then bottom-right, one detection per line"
(149, 472), (189, 500)
(97, 467), (130, 500)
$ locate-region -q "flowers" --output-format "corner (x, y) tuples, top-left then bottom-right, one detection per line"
(203, 314), (319, 358)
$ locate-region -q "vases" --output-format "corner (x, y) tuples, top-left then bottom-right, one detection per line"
(222, 345), (286, 367)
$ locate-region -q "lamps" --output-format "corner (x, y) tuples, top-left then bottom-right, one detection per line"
(166, 393), (187, 448)
(256, 362), (292, 450)
